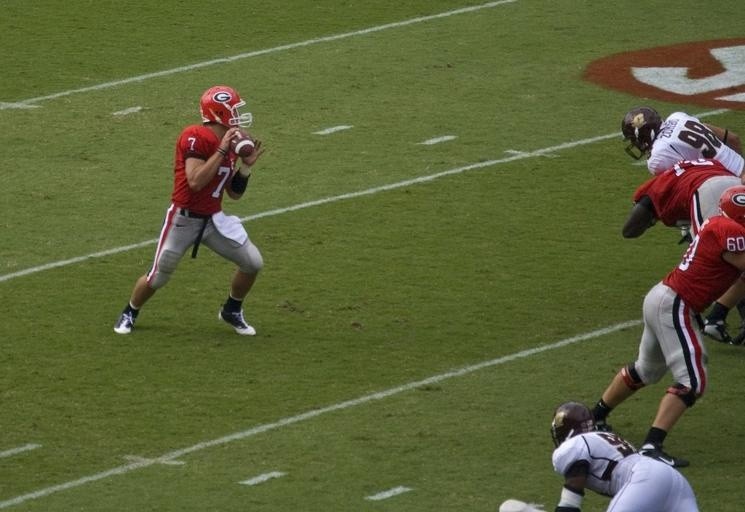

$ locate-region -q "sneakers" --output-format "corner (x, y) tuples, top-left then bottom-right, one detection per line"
(595, 420), (613, 432)
(638, 440), (689, 468)
(218, 308), (256, 336)
(113, 312), (136, 335)
(699, 316), (732, 343)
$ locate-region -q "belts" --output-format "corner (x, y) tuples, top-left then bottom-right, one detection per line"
(180, 210), (212, 219)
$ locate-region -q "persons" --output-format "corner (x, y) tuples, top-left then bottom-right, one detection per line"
(622, 107), (745, 346)
(590, 185), (745, 467)
(551, 402), (699, 512)
(113, 85), (266, 335)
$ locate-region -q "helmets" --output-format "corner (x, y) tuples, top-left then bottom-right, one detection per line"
(199, 86), (253, 129)
(718, 185), (745, 225)
(550, 401), (595, 447)
(621, 106), (662, 161)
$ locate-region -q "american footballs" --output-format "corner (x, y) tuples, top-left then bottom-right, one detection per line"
(230, 128), (255, 157)
